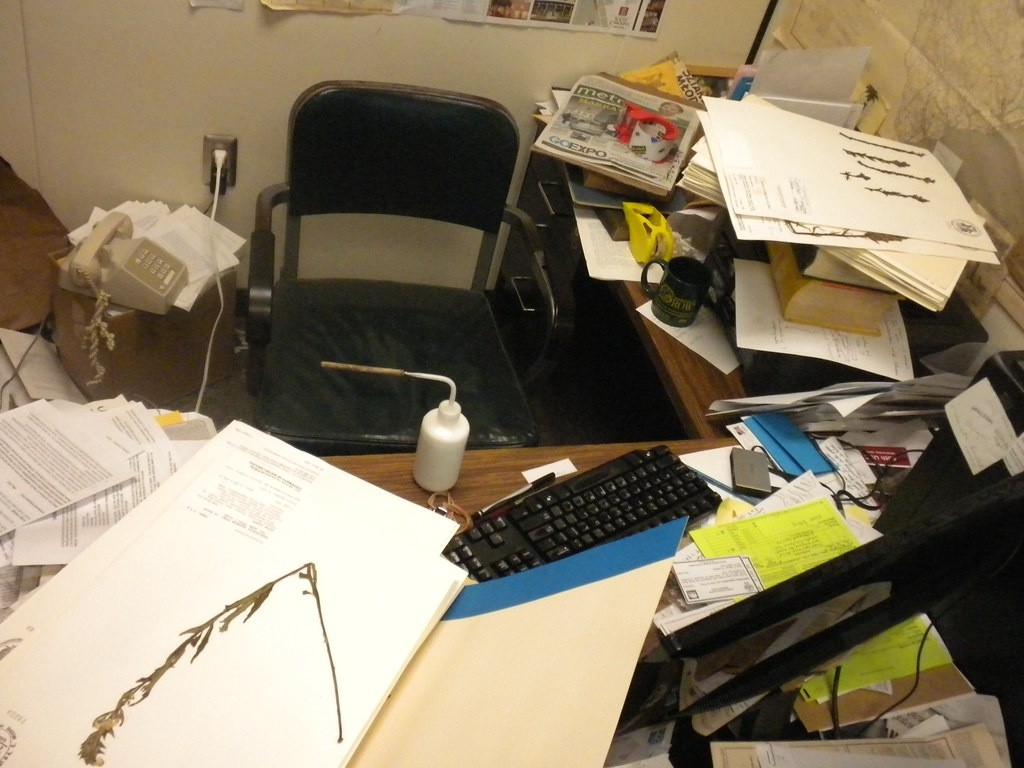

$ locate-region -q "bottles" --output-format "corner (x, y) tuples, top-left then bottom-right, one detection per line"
(412, 400), (470, 493)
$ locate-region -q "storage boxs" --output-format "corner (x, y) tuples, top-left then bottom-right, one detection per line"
(47, 247), (239, 409)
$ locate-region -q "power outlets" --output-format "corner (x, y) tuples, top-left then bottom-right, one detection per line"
(203, 134), (238, 187)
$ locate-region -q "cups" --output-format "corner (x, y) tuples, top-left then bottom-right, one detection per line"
(641, 257), (714, 327)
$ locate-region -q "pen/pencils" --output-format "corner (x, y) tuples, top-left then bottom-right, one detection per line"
(472, 472), (556, 524)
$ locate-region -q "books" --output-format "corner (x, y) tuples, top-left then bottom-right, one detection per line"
(788, 404), (949, 432)
(530, 76), (704, 197)
(676, 95), (986, 338)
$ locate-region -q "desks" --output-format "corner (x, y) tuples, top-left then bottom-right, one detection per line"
(319, 439), (741, 661)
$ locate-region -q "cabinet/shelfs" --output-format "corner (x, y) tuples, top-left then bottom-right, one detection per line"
(489, 108), (747, 440)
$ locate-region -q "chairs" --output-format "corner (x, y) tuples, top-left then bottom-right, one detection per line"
(246, 80), (578, 456)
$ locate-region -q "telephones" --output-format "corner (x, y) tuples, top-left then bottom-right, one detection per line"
(55, 211), (191, 318)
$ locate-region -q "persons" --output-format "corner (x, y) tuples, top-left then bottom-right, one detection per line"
(658, 100), (683, 118)
